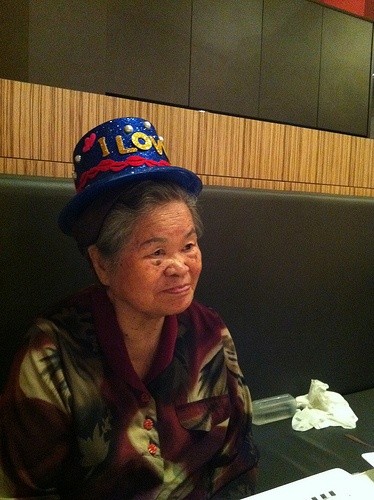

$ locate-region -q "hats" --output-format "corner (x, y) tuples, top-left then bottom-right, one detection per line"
(55, 114), (204, 235)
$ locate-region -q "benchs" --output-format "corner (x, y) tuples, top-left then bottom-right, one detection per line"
(0, 171), (373, 456)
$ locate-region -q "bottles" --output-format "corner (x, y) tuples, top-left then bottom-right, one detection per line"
(252, 393), (297, 426)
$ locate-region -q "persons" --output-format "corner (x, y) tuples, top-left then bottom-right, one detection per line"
(1, 174), (261, 500)
